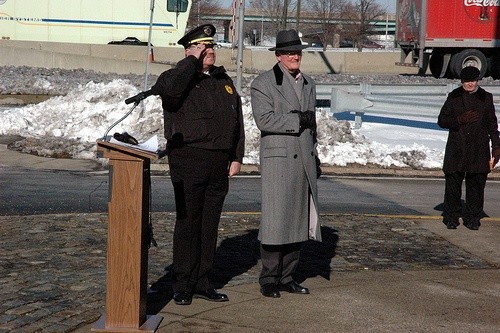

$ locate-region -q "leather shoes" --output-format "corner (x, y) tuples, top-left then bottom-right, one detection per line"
(260, 288), (280, 298)
(278, 281), (309, 294)
(173, 292), (192, 305)
(193, 289), (230, 301)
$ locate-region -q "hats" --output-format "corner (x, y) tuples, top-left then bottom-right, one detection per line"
(178, 24), (217, 48)
(461, 66), (482, 83)
(268, 29), (309, 50)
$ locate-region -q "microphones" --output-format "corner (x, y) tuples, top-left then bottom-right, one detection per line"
(124, 83), (163, 104)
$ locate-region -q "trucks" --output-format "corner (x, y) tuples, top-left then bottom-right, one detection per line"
(393, 0), (500, 81)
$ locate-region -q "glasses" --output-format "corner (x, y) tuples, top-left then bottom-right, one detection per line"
(280, 51), (304, 56)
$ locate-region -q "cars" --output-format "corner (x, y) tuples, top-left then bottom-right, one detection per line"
(214, 38), (236, 49)
(107, 35), (151, 46)
(313, 32), (386, 50)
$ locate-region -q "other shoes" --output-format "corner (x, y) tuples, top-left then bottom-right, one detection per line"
(447, 222), (456, 229)
(463, 221), (478, 230)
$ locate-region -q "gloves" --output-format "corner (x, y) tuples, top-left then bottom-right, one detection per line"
(456, 111), (480, 125)
(315, 155), (322, 179)
(292, 109), (315, 136)
(492, 143), (500, 168)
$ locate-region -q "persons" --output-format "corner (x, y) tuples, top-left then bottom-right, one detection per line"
(437, 65), (500, 230)
(156, 24), (245, 304)
(250, 28), (322, 297)
